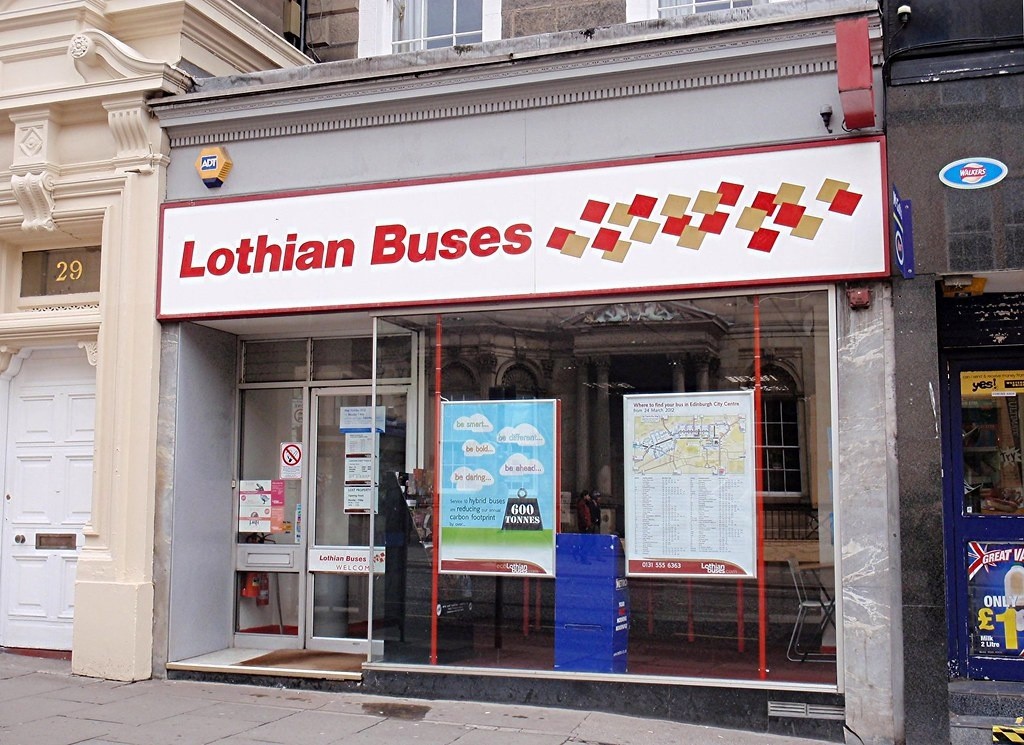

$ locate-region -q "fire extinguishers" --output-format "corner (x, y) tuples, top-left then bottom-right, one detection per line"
(256, 533), (276, 606)
(241, 532), (262, 599)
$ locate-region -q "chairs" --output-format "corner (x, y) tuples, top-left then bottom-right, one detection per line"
(785, 556), (835, 663)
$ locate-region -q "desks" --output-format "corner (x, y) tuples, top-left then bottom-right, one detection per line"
(798, 561), (836, 662)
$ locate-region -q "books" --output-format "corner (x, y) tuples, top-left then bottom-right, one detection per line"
(394, 468), (434, 550)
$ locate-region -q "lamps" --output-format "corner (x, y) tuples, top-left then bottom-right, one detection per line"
(889, 5), (911, 45)
(819, 104), (833, 133)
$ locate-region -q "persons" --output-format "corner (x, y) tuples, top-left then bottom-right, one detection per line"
(576, 490), (594, 535)
(589, 491), (603, 535)
